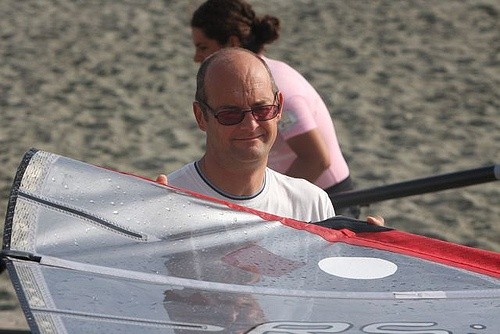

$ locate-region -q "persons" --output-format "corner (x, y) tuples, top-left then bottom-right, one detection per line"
(189, 0), (354, 214)
(155, 44), (385, 231)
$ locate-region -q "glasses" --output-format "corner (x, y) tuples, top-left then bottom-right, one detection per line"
(193, 90), (280, 126)
